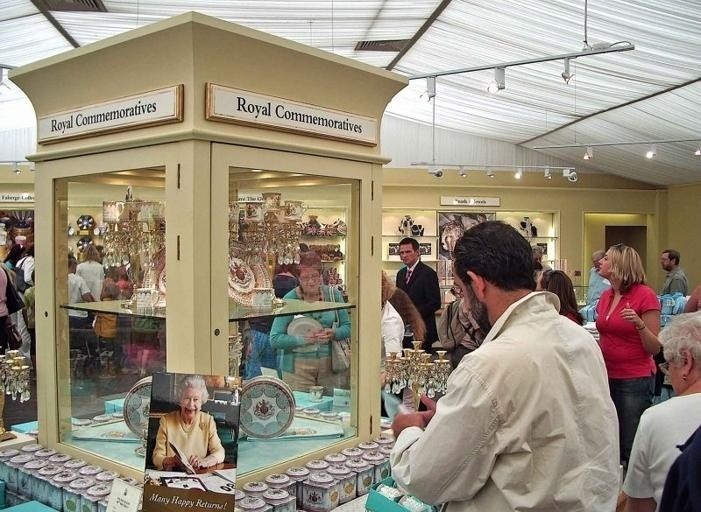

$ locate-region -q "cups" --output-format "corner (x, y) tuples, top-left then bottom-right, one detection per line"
(135, 287), (152, 311)
(310, 386), (324, 400)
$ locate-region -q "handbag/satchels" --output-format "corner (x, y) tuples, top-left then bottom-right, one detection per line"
(328, 284), (352, 375)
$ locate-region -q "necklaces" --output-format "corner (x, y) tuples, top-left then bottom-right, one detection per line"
(300, 287), (327, 320)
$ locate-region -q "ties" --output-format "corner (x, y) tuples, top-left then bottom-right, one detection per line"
(403, 267), (415, 286)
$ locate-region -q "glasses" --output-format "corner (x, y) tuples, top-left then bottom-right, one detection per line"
(657, 360), (670, 374)
(299, 273), (322, 282)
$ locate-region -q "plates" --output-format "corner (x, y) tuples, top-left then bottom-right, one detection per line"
(228, 247), (273, 305)
(144, 247), (167, 308)
(286, 317), (324, 338)
(134, 446), (146, 458)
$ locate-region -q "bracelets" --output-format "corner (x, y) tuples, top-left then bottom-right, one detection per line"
(635, 321), (645, 331)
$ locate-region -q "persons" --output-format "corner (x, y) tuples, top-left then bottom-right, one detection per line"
(528, 245), (552, 292)
(64, 257), (96, 330)
(244, 330), (278, 369)
(270, 251), (352, 392)
(4, 244), (24, 269)
(15, 245), (38, 286)
(130, 315), (160, 380)
(540, 271), (585, 325)
(619, 309), (701, 511)
(661, 249), (688, 296)
(152, 376), (225, 468)
(76, 246), (105, 302)
(380, 270), (430, 398)
(442, 295), (483, 373)
(0, 264), (25, 356)
(273, 263), (298, 297)
(391, 222), (620, 512)
(22, 268), (41, 382)
(584, 250), (611, 306)
(95, 278), (122, 379)
(397, 238), (441, 354)
(596, 243), (662, 462)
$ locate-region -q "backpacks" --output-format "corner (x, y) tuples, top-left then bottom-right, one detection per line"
(437, 299), (470, 349)
